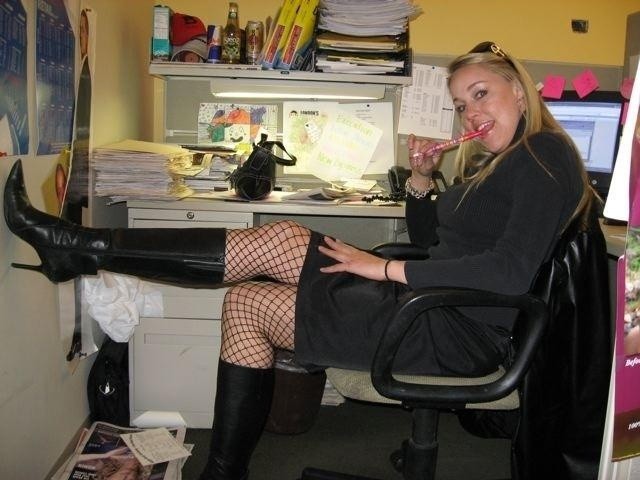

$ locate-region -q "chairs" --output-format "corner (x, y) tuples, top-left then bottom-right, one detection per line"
(300, 183), (604, 479)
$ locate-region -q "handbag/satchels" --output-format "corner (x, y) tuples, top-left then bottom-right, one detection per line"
(231, 133), (297, 200)
(89, 332), (130, 430)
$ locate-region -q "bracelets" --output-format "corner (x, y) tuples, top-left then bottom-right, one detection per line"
(384, 257), (398, 283)
(403, 178), (436, 201)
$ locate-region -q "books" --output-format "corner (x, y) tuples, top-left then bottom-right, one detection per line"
(264, 1), (405, 75)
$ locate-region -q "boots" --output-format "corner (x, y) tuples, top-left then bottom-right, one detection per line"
(195, 357), (276, 480)
(3, 157), (227, 290)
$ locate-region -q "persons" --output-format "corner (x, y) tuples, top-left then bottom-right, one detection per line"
(3, 43), (586, 479)
(55, 8), (91, 361)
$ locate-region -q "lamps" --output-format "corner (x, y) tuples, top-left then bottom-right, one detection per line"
(210, 78), (385, 100)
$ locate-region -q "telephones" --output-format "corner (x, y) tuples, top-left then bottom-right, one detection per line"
(386, 164), (448, 202)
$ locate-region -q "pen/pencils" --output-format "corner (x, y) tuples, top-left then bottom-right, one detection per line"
(411, 130), (488, 159)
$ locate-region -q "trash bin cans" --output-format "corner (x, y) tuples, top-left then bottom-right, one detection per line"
(266, 349), (327, 437)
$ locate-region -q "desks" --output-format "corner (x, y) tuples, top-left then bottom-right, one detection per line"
(120, 57), (627, 434)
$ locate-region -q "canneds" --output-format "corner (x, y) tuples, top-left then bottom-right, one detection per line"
(245, 21), (264, 63)
(207, 25), (224, 63)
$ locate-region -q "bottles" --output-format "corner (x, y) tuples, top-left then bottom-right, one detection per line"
(221, 3), (243, 62)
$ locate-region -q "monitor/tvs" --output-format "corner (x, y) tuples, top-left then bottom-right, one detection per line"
(537, 90), (630, 192)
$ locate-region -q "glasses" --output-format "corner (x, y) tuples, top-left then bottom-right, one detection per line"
(467, 41), (520, 75)
